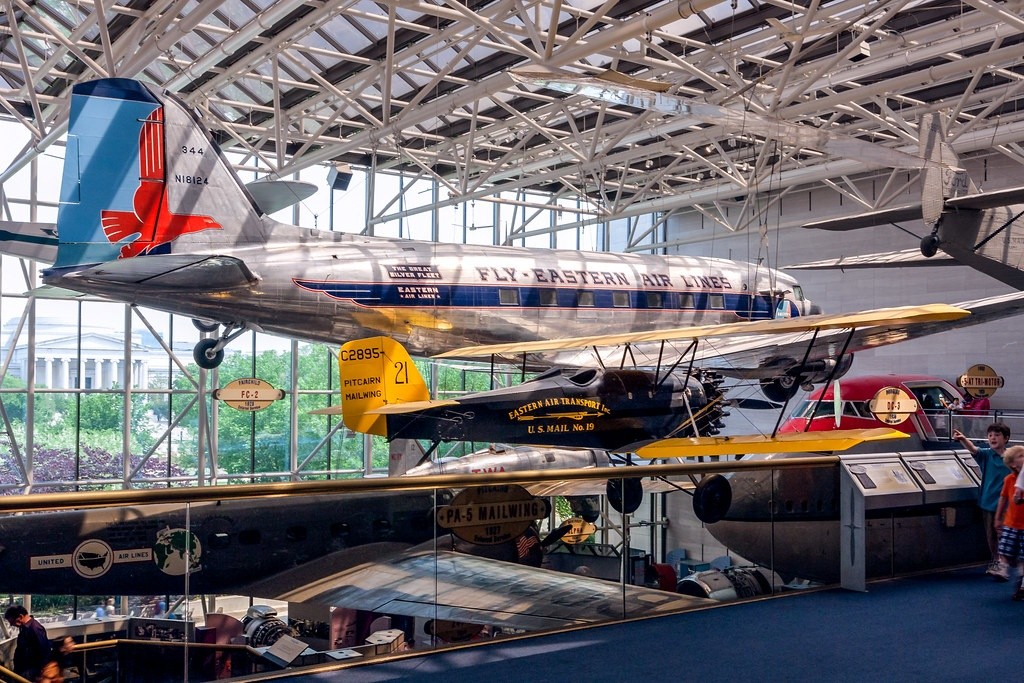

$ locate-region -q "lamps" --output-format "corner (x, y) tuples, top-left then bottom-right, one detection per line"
(845, 29), (871, 62)
(326, 164), (352, 192)
(645, 138), (749, 180)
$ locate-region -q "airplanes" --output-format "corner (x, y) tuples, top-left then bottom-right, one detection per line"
(1, 63), (1024, 589)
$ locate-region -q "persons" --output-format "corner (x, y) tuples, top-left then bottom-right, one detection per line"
(106, 598), (115, 616)
(4, 606), (51, 683)
(40, 635), (80, 683)
(1013, 464), (1024, 601)
(95, 601), (105, 617)
(948, 398), (963, 415)
(158, 596), (166, 612)
(952, 422), (1012, 574)
(989, 445), (1024, 581)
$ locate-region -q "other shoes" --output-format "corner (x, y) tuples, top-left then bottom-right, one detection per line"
(1014, 588), (1024, 601)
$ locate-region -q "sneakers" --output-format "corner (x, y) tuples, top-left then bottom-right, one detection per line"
(985, 558), (1011, 582)
(1015, 566), (1024, 578)
